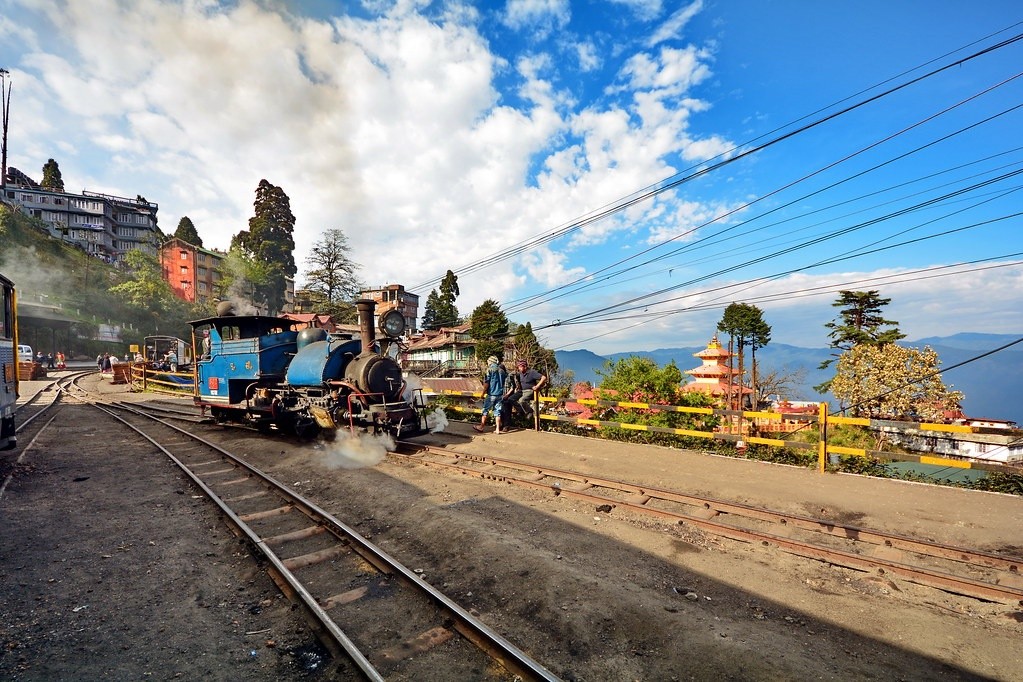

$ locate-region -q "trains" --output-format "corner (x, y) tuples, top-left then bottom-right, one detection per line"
(0, 273), (21, 451)
(183, 300), (430, 454)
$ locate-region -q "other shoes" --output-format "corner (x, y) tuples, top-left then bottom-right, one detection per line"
(502, 426), (510, 432)
(527, 409), (533, 420)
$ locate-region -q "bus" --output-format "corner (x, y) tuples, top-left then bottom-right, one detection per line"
(144, 335), (193, 366)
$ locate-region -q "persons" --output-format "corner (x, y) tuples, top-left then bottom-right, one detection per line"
(164, 351), (177, 373)
(201, 329), (212, 359)
(37, 351), (44, 358)
(47, 352), (54, 368)
(135, 352), (142, 358)
(55, 351), (66, 370)
(125, 352), (130, 362)
(96, 352), (119, 374)
(473, 354), (546, 435)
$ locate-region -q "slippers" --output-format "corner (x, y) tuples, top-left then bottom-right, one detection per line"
(492, 431), (500, 435)
(473, 425), (483, 432)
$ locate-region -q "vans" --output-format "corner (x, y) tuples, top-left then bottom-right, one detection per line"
(18, 345), (33, 361)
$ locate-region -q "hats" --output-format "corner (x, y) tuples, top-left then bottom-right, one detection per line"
(488, 356), (498, 364)
